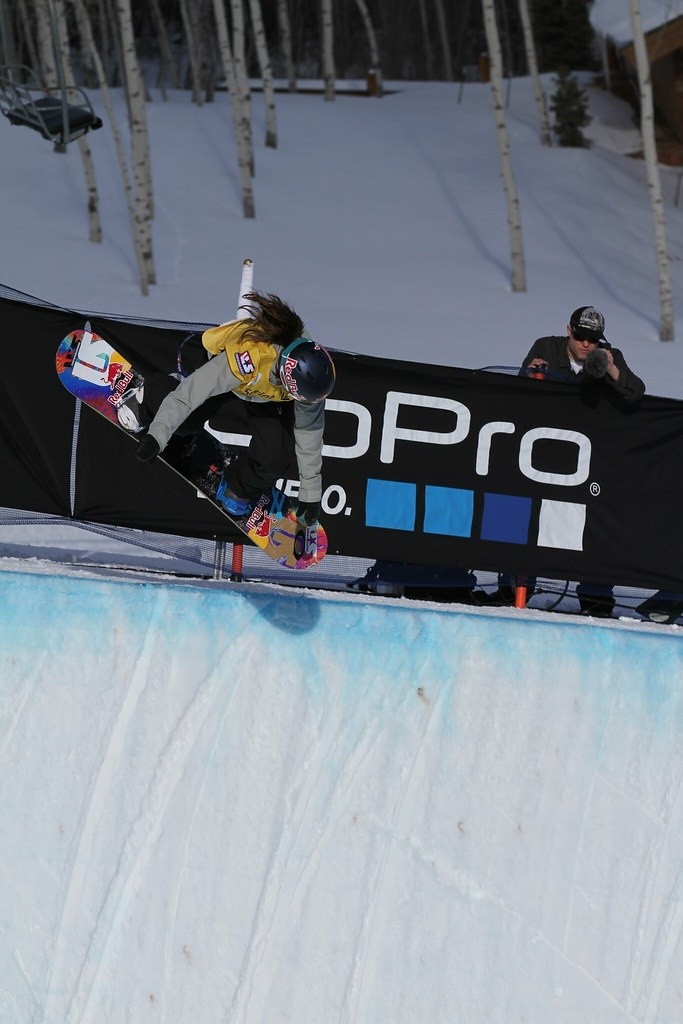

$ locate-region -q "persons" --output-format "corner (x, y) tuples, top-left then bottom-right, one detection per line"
(516, 304), (647, 618)
(135, 287), (336, 530)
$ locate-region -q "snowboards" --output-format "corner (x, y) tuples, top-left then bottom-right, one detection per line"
(55, 328), (328, 570)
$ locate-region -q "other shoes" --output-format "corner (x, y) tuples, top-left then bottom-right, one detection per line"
(489, 588), (516, 603)
(116, 385), (147, 434)
(581, 603), (613, 618)
(221, 481), (247, 515)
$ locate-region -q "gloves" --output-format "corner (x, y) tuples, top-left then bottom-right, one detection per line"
(137, 436), (160, 460)
(296, 499), (322, 527)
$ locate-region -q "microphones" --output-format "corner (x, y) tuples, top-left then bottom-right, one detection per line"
(585, 349), (608, 379)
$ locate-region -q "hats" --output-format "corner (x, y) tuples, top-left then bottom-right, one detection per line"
(569, 305), (605, 342)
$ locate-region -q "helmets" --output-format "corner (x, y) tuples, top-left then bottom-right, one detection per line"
(279, 339), (334, 403)
